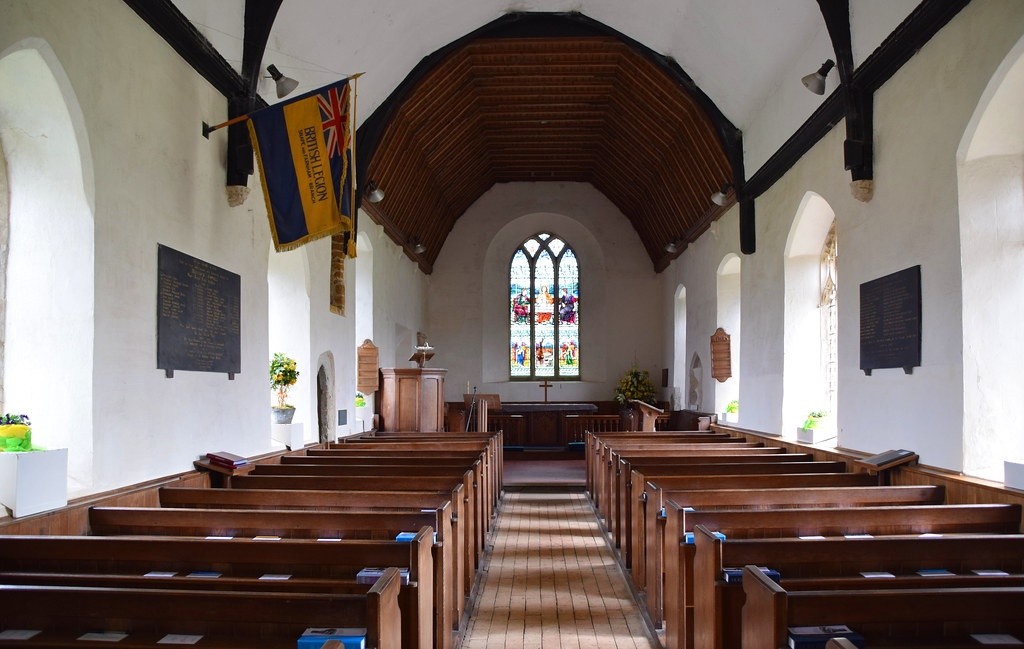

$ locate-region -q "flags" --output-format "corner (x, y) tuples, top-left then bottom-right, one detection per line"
(250, 76), (363, 256)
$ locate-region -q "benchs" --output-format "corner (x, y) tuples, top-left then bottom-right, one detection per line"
(0, 430), (504, 649)
(585, 429), (1024, 649)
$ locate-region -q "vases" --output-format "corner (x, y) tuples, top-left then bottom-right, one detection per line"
(272, 405), (295, 424)
(0, 424), (32, 452)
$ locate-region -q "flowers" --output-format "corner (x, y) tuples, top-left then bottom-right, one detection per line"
(0, 414), (33, 425)
(269, 352), (300, 405)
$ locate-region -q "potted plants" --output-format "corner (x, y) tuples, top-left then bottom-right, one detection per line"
(722, 400), (738, 422)
(356, 393), (364, 406)
(796, 411), (830, 444)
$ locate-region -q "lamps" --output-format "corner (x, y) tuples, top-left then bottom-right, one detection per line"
(711, 182), (733, 206)
(259, 63), (299, 99)
(801, 59), (836, 96)
(406, 236), (427, 254)
(364, 180), (385, 203)
(666, 235), (682, 252)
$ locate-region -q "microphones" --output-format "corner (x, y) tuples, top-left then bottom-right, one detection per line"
(474, 387), (476, 391)
